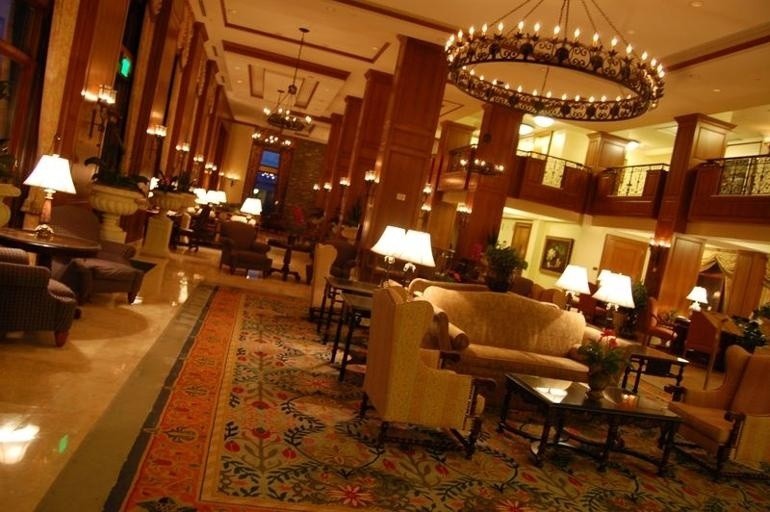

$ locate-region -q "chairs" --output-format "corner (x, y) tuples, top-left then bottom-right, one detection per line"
(310, 216), (768, 384)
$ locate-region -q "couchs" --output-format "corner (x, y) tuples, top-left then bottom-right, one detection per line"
(664, 342), (768, 484)
(408, 284), (591, 401)
(0, 141), (275, 348)
(354, 284), (487, 461)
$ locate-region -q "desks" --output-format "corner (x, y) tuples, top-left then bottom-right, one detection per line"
(621, 337), (685, 411)
(266, 238), (313, 283)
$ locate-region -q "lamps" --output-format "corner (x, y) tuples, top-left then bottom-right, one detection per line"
(443, 0), (668, 126)
(262, 25), (317, 133)
(306, 154), (506, 228)
(80, 77), (120, 152)
(147, 123), (243, 191)
(248, 84), (295, 153)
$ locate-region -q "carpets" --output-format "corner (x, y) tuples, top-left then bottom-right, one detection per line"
(36, 278), (770, 512)
